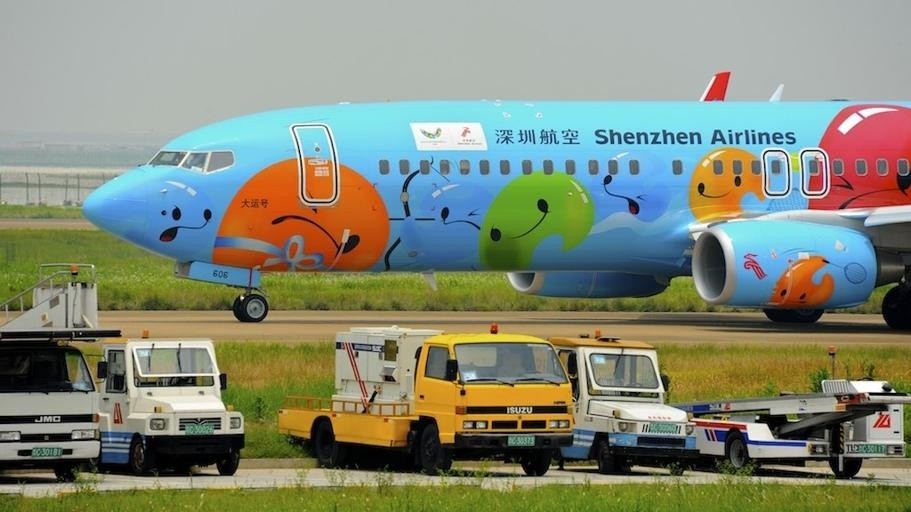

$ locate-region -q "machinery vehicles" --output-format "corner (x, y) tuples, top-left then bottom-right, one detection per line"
(668, 349), (909, 477)
(0, 263), (121, 483)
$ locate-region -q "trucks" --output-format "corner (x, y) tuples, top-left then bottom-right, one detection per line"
(277, 320), (577, 476)
(543, 328), (699, 475)
(72, 336), (247, 475)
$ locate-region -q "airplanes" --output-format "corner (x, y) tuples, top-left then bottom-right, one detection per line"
(79, 68), (911, 344)
